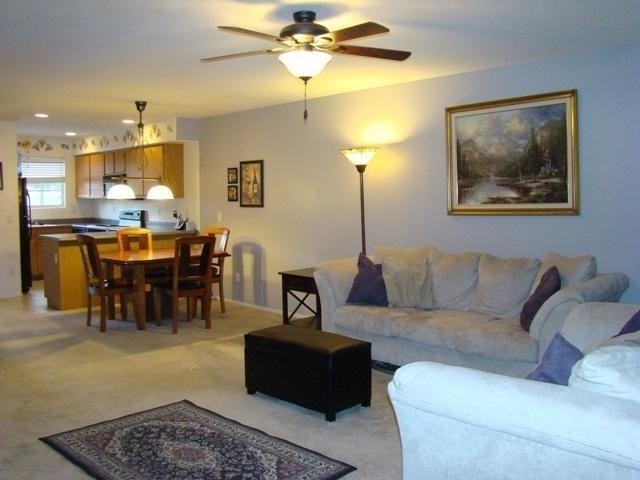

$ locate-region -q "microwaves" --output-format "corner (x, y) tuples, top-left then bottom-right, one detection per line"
(103, 173), (126, 199)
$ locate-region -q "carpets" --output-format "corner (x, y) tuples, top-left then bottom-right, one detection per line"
(38, 396), (358, 480)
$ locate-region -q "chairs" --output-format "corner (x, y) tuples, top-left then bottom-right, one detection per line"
(166, 225), (231, 313)
(117, 226), (162, 321)
(150, 235), (213, 332)
(74, 232), (138, 331)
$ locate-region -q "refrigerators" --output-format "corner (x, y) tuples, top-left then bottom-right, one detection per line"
(18, 177), (34, 295)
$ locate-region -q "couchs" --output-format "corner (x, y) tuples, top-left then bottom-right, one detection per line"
(386, 301), (639, 479)
(312, 254), (629, 378)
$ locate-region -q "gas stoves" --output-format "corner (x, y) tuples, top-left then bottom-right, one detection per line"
(87, 224), (140, 234)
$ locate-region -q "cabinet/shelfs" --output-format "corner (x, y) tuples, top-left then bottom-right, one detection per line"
(29, 226), (73, 281)
(104, 150), (126, 174)
(75, 151), (105, 200)
(126, 144), (186, 199)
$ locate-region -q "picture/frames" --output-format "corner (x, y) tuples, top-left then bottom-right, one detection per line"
(443, 89), (582, 218)
(0, 162), (3, 190)
(228, 185), (239, 201)
(227, 167), (238, 184)
(239, 159), (265, 209)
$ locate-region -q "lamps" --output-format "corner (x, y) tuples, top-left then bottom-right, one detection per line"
(341, 146), (383, 254)
(277, 42), (330, 120)
(102, 101), (177, 200)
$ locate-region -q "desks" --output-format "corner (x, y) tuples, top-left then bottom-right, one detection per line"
(279, 266), (322, 331)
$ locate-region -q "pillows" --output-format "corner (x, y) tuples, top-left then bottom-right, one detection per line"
(345, 244), (597, 332)
(567, 342), (639, 400)
(526, 330), (582, 387)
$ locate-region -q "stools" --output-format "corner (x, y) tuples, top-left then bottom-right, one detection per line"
(243, 326), (373, 422)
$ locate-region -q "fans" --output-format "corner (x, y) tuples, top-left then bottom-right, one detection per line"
(199, 9), (413, 63)
